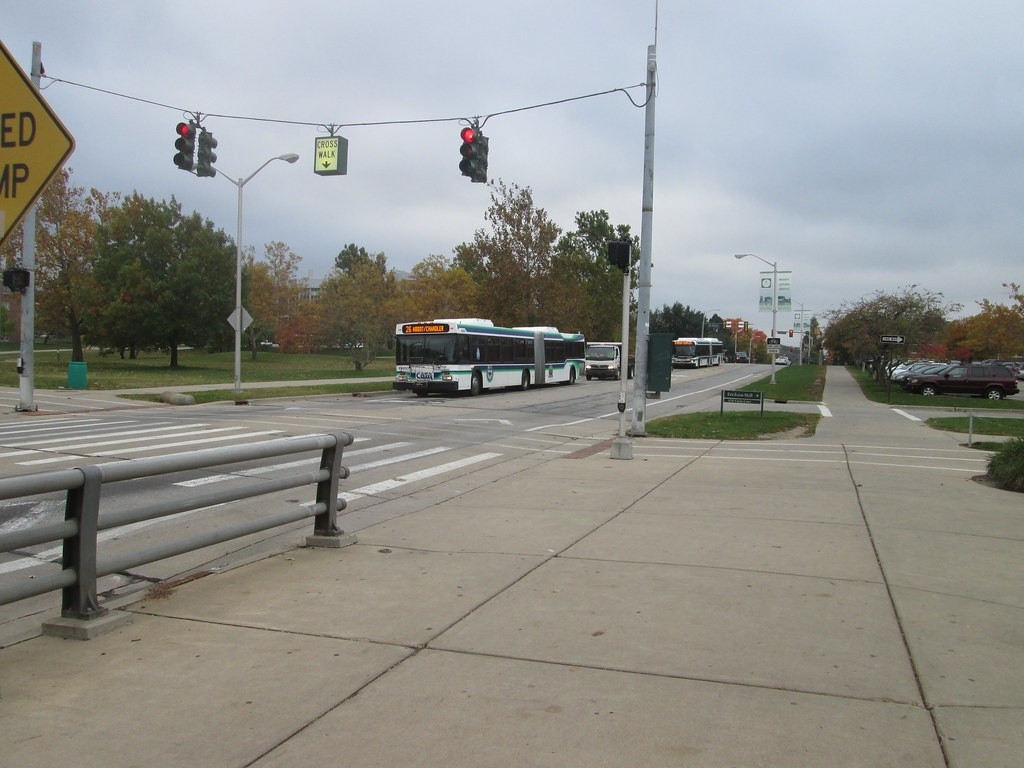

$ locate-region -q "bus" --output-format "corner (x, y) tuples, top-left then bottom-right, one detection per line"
(388, 318), (587, 397)
(671, 338), (724, 369)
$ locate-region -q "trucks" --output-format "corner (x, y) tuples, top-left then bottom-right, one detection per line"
(586, 342), (635, 380)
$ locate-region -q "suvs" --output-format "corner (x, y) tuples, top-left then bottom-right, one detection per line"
(905, 364), (1020, 400)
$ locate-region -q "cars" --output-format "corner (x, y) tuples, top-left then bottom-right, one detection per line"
(775, 356), (791, 365)
(869, 358), (1024, 391)
(723, 356), (728, 363)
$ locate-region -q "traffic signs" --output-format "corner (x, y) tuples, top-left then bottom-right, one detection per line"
(313, 134), (348, 177)
(880, 335), (905, 345)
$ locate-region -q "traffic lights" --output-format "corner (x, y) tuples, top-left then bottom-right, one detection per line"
(172, 122), (196, 170)
(458, 127), (478, 177)
(197, 131), (218, 177)
(789, 330), (793, 337)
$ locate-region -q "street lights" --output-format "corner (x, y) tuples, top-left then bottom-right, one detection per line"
(178, 152), (300, 406)
(702, 309), (720, 338)
(734, 252), (777, 385)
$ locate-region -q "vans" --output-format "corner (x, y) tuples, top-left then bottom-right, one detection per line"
(736, 352), (748, 363)
